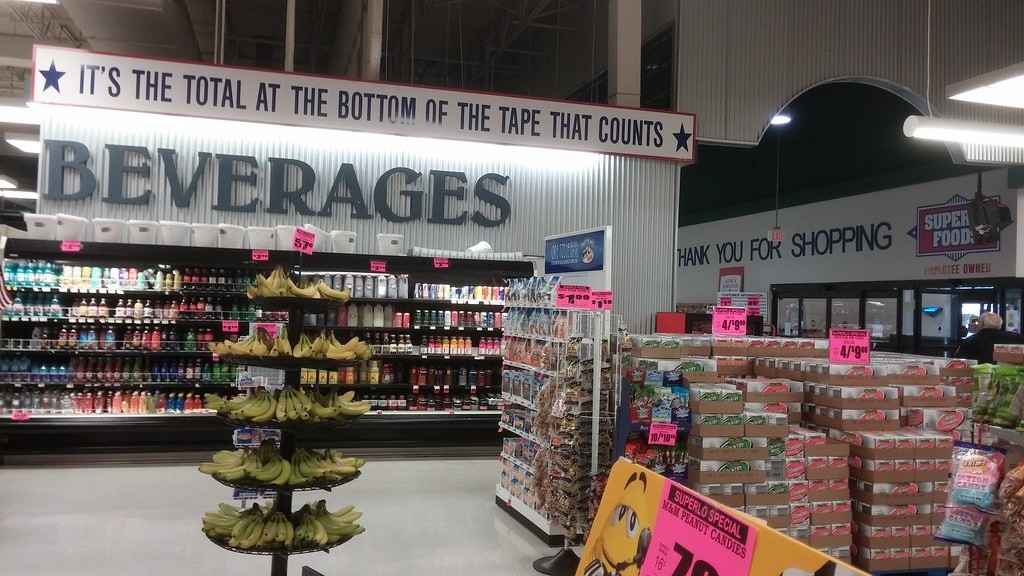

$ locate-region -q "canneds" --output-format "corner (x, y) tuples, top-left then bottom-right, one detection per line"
(414, 309), (507, 329)
(393, 312), (410, 328)
(414, 282), (508, 302)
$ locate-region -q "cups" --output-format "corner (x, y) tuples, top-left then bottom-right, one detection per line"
(403, 313), (410, 327)
(303, 313), (316, 326)
(317, 313), (326, 325)
(393, 313), (402, 327)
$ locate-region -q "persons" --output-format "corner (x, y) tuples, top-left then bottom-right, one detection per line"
(954, 312), (1005, 366)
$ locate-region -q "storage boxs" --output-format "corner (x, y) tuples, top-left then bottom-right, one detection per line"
(629, 331), (978, 576)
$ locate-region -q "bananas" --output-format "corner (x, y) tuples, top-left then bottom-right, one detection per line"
(201, 499), (366, 550)
(246, 265), (351, 303)
(203, 385), (372, 422)
(199, 438), (366, 485)
(207, 326), (373, 361)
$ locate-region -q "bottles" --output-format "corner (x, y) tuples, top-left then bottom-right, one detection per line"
(0, 388), (202, 414)
(313, 273), (409, 299)
(32, 325), (239, 351)
(300, 361), (394, 383)
(414, 310), (508, 328)
(419, 335), (507, 356)
(409, 367), (492, 388)
(414, 283), (509, 300)
(350, 332), (413, 355)
(327, 302), (392, 327)
(0, 259), (261, 291)
(362, 393), (504, 411)
(0, 355), (239, 382)
(0, 294), (289, 320)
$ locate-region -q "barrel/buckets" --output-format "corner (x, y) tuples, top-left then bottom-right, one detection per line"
(23, 212), (57, 240)
(376, 234), (404, 256)
(56, 213), (89, 241)
(465, 240), (494, 253)
(93, 218), (357, 253)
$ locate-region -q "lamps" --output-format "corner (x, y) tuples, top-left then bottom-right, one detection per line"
(902, 0), (1024, 147)
